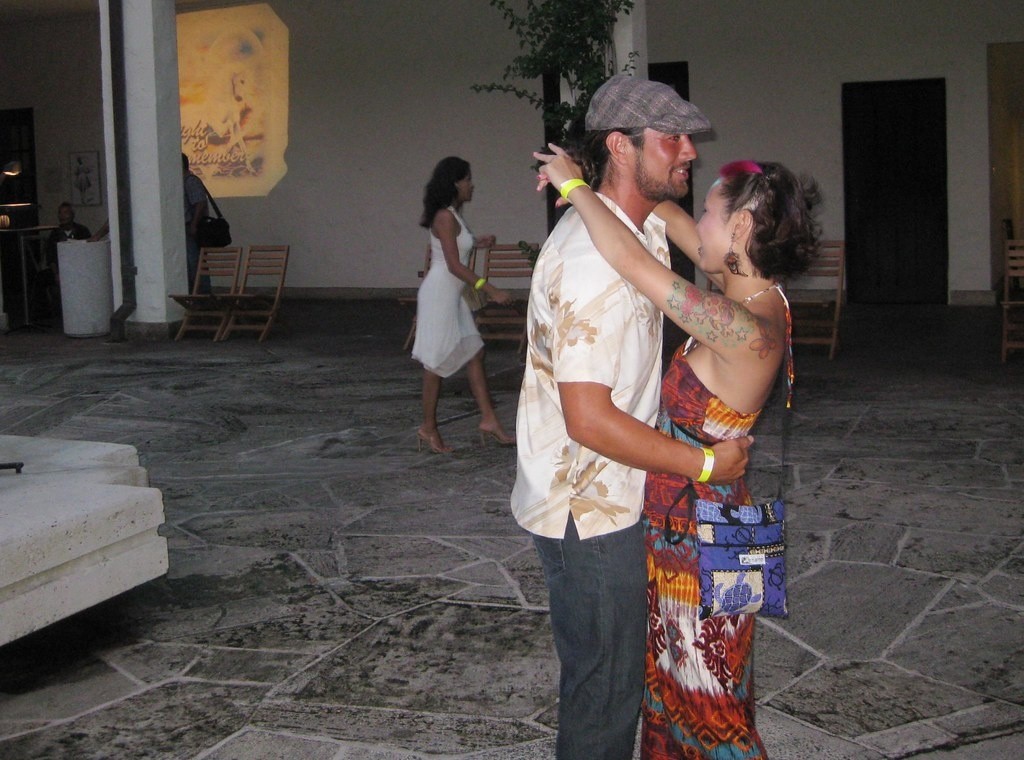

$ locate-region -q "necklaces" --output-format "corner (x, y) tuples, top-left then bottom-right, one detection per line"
(681, 280), (795, 409)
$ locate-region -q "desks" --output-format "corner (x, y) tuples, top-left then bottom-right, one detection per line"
(0, 222), (54, 335)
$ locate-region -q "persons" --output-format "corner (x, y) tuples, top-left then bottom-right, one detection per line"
(87, 218), (109, 242)
(533, 143), (821, 760)
(182, 152), (212, 304)
(31, 202), (91, 322)
(508, 75), (713, 760)
(411, 156), (516, 453)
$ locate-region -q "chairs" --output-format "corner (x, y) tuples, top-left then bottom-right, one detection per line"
(164, 243), (291, 344)
(702, 238), (845, 363)
(1000, 239), (1024, 363)
(402, 240), (541, 352)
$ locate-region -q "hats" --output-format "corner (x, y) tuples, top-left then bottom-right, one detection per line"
(584, 74), (713, 135)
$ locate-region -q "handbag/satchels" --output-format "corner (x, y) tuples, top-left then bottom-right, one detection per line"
(696, 500), (789, 620)
(461, 286), (487, 312)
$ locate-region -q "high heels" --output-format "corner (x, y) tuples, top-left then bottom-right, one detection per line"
(478, 427), (515, 446)
(416, 434), (455, 454)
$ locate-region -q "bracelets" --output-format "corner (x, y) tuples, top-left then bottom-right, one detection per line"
(560, 178), (591, 200)
(474, 278), (486, 290)
